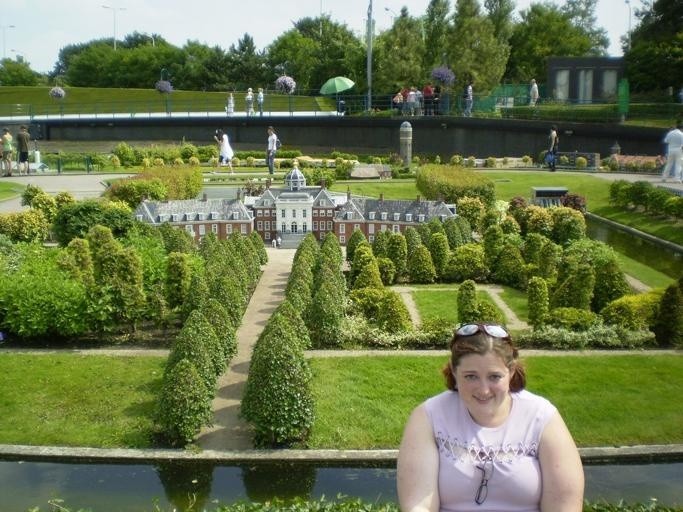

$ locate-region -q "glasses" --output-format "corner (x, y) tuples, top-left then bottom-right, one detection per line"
(450, 323), (513, 349)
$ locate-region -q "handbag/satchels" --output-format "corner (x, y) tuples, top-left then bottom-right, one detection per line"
(277, 139), (282, 149)
(545, 152), (554, 162)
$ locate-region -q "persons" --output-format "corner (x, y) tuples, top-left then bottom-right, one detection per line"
(224, 92), (234, 115)
(275, 231), (282, 248)
(397, 320), (585, 511)
(660, 120), (682, 183)
(209, 128), (235, 176)
(319, 76), (355, 99)
(270, 232), (277, 249)
(337, 96), (351, 116)
(464, 81), (475, 118)
(266, 126), (279, 176)
(0, 127), (12, 177)
(256, 88), (263, 116)
(544, 125), (559, 172)
(391, 81), (441, 117)
(245, 87), (255, 116)
(529, 79), (539, 107)
(15, 125), (29, 176)
